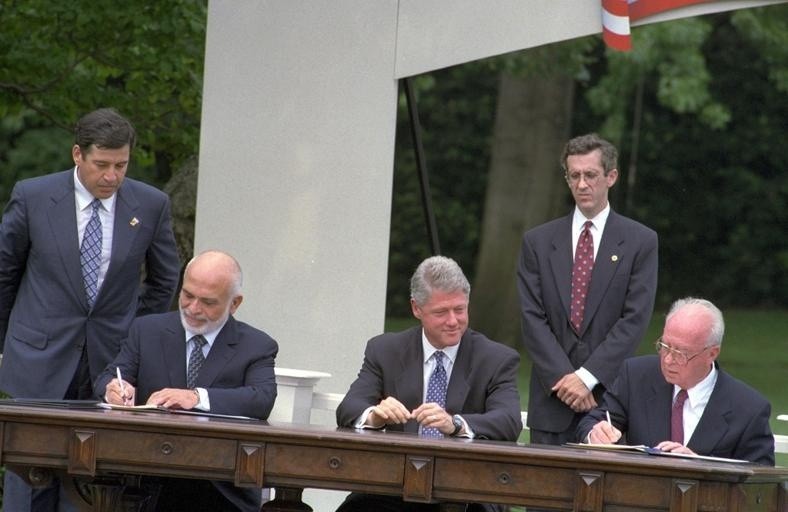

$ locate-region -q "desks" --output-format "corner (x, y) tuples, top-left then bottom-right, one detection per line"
(0, 400), (788, 512)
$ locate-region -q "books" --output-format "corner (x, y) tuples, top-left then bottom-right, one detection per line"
(560, 441), (749, 464)
(0, 397), (103, 410)
(96, 401), (260, 421)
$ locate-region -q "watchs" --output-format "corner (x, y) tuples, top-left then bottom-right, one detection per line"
(194, 387), (202, 411)
(447, 417), (465, 437)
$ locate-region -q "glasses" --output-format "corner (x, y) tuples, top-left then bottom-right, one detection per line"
(654, 336), (709, 367)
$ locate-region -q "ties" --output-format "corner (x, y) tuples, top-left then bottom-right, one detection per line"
(668, 388), (689, 447)
(421, 351), (447, 444)
(186, 334), (208, 392)
(79, 197), (104, 312)
(568, 220), (595, 335)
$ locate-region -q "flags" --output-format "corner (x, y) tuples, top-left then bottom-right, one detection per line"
(601, 0), (712, 53)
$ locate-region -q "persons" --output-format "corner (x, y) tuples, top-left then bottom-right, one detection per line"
(518, 134), (660, 447)
(0, 106), (184, 511)
(574, 297), (775, 470)
(337, 254), (519, 511)
(90, 251), (277, 511)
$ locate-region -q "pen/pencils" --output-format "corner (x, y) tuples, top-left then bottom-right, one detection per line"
(606, 411), (612, 428)
(116, 367), (127, 406)
